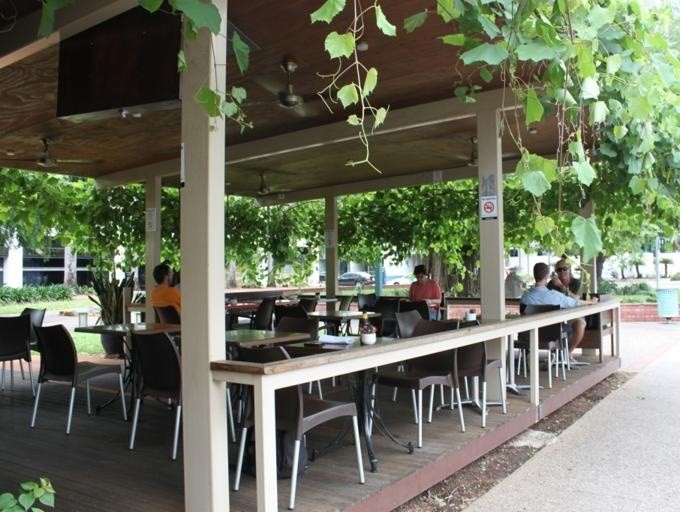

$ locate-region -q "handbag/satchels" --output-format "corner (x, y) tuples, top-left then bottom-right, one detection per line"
(240, 425), (308, 479)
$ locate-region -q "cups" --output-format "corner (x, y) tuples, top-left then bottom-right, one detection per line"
(361, 332), (377, 345)
(465, 311), (476, 321)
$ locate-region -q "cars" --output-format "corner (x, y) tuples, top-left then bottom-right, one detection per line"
(392, 273), (419, 287)
(341, 270), (374, 289)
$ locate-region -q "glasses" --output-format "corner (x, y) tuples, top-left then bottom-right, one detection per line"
(556, 267), (569, 273)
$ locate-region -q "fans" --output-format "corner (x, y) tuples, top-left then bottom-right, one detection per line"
(437, 137), (519, 170)
(232, 60), (341, 120)
(227, 167), (295, 198)
(2, 137), (104, 170)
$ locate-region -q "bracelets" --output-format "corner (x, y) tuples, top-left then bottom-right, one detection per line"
(558, 283), (564, 291)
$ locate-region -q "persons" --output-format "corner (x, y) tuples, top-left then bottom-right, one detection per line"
(547, 258), (587, 364)
(398, 264), (443, 321)
(518, 261), (599, 338)
(149, 265), (180, 318)
